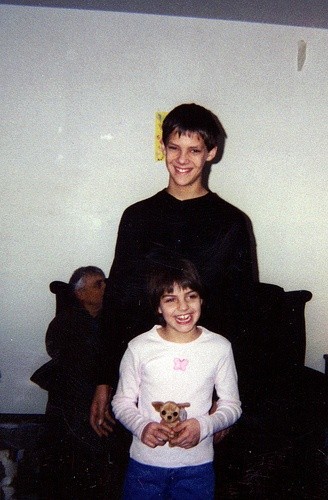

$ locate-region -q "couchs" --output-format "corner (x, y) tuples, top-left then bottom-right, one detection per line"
(31, 277), (312, 422)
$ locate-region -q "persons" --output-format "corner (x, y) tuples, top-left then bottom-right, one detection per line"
(90, 103), (266, 500)
(111, 259), (242, 500)
(45, 267), (124, 500)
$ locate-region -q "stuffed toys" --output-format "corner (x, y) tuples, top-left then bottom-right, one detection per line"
(151, 401), (190, 447)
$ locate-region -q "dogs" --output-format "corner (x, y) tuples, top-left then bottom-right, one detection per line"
(151, 401), (190, 448)
